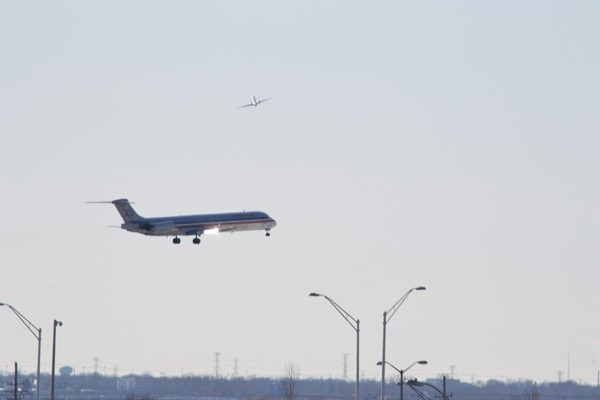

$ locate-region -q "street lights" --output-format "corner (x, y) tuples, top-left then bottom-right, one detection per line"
(376, 360), (428, 399)
(405, 374), (453, 400)
(396, 381), (431, 400)
(380, 286), (426, 399)
(308, 292), (360, 399)
(0, 303), (41, 400)
(51, 319), (63, 399)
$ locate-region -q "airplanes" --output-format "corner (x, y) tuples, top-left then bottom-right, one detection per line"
(238, 95), (272, 108)
(113, 198), (277, 245)
